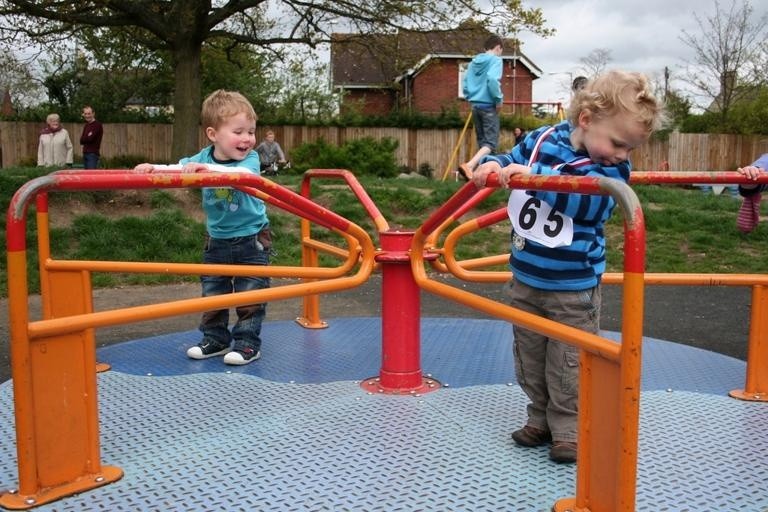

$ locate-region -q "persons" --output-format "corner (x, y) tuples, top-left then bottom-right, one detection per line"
(457, 36), (504, 182)
(36, 113), (75, 169)
(254, 130), (287, 177)
(569, 75), (589, 95)
(131, 88), (273, 365)
(78, 103), (103, 169)
(469, 68), (661, 462)
(735, 152), (768, 198)
(512, 126), (529, 146)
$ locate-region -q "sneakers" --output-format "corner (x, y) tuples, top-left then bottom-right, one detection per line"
(549, 446), (578, 463)
(458, 163), (474, 181)
(187, 343), (230, 359)
(224, 348), (260, 365)
(512, 425), (551, 447)
